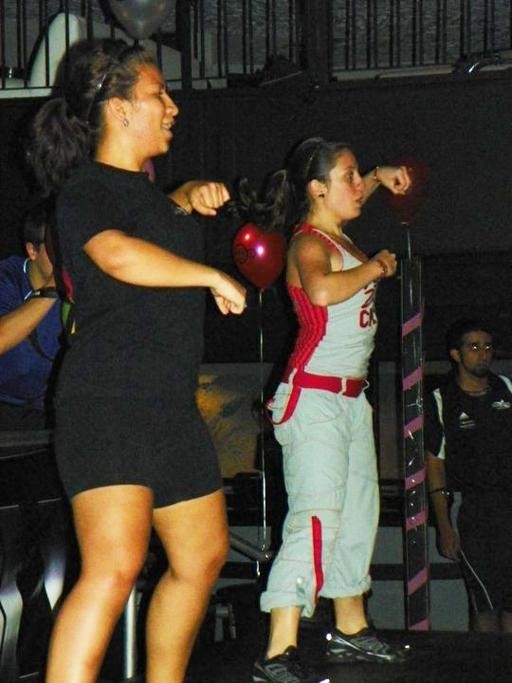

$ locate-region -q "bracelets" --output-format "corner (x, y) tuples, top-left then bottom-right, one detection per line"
(371, 257), (390, 281)
(36, 287), (59, 299)
(373, 163), (384, 186)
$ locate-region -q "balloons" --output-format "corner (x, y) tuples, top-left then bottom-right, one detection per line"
(109, 0), (176, 37)
(384, 153), (432, 225)
(233, 221), (289, 291)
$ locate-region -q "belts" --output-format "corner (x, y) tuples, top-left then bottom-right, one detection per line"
(278, 365), (370, 398)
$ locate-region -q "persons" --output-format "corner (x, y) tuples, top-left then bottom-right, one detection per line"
(227, 137), (413, 683)
(29, 37), (246, 683)
(0, 212), (61, 429)
(423, 320), (512, 636)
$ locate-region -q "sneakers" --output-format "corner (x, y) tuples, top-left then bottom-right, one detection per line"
(325, 624), (406, 662)
(253, 646), (330, 683)
